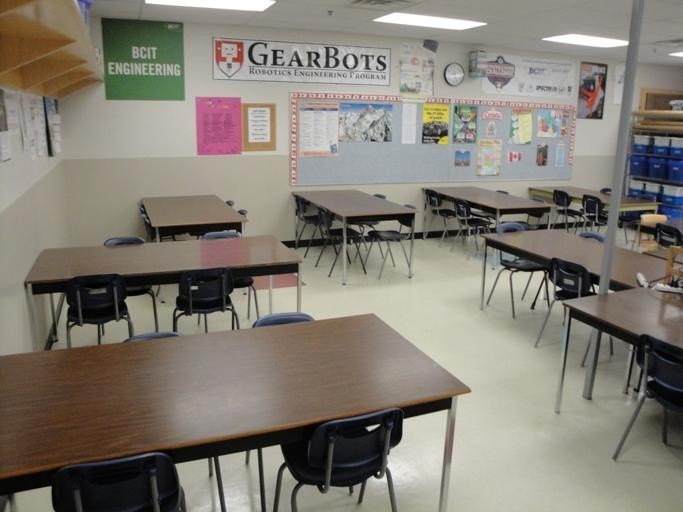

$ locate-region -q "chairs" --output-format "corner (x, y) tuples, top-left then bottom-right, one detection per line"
(364, 204), (416, 280)
(197, 232), (261, 331)
(535, 257), (615, 367)
(245, 312), (318, 512)
(102, 236), (160, 338)
(120, 334), (224, 511)
(274, 406), (407, 511)
(65, 273), (135, 346)
(612, 334), (683, 462)
(422, 185), (544, 259)
(550, 185), (682, 249)
(345, 194), (388, 261)
(294, 194), (339, 257)
(487, 222), (550, 320)
(140, 206), (191, 241)
(52, 452), (189, 512)
(173, 268), (241, 333)
(531, 230), (605, 326)
(313, 205), (368, 278)
(188, 200), (249, 241)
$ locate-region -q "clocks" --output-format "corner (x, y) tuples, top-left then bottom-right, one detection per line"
(443, 62), (466, 87)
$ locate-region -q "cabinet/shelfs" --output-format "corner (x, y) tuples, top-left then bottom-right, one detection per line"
(618, 109), (683, 228)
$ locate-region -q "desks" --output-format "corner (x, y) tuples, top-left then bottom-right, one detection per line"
(421, 187), (550, 265)
(480, 232), (680, 312)
(554, 286), (683, 415)
(3, 313), (473, 511)
(528, 186), (663, 249)
(142, 193), (248, 242)
(291, 190), (420, 285)
(24, 234), (302, 342)
